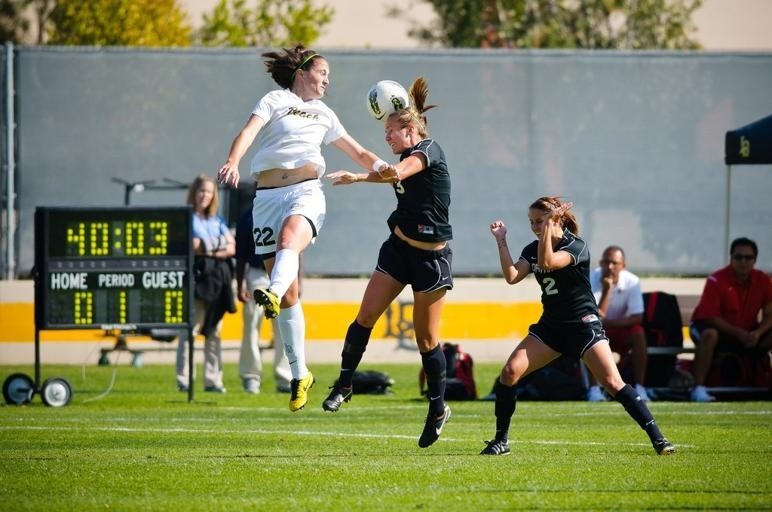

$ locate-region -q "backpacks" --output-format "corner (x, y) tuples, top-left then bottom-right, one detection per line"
(419, 343), (476, 400)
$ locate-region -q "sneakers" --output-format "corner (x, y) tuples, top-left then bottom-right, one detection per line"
(653, 438), (674, 455)
(692, 386), (715, 402)
(588, 386), (607, 401)
(633, 383), (650, 401)
(253, 289), (279, 319)
(480, 440), (510, 456)
(323, 384), (352, 411)
(178, 372), (292, 392)
(289, 372), (314, 411)
(419, 403), (450, 448)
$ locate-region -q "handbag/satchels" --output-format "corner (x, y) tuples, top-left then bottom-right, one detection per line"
(335, 371), (392, 393)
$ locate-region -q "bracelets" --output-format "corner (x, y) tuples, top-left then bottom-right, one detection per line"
(497, 238), (508, 248)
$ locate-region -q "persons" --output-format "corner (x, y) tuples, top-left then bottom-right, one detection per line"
(477, 195), (674, 455)
(176, 174), (237, 394)
(216, 42), (401, 409)
(235, 178), (292, 395)
(581, 244), (652, 402)
(687, 237), (771, 402)
(324, 75), (454, 448)
(373, 157), (388, 172)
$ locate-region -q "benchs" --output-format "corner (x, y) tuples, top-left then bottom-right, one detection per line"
(581, 345), (772, 395)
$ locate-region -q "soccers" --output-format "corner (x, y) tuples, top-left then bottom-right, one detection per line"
(366, 79), (407, 120)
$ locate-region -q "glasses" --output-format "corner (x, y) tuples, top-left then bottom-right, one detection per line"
(732, 254), (755, 261)
